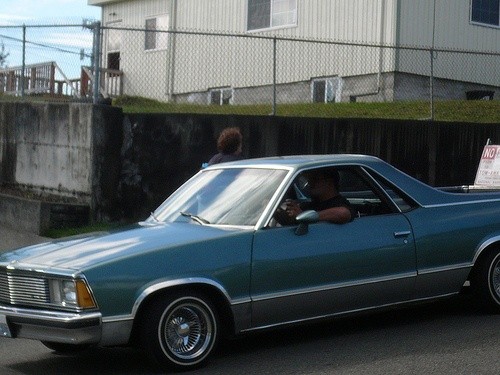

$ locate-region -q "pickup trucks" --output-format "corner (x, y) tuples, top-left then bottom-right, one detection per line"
(0, 153), (500, 372)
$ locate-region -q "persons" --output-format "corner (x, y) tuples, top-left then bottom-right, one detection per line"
(285, 166), (356, 226)
(199, 127), (243, 169)
